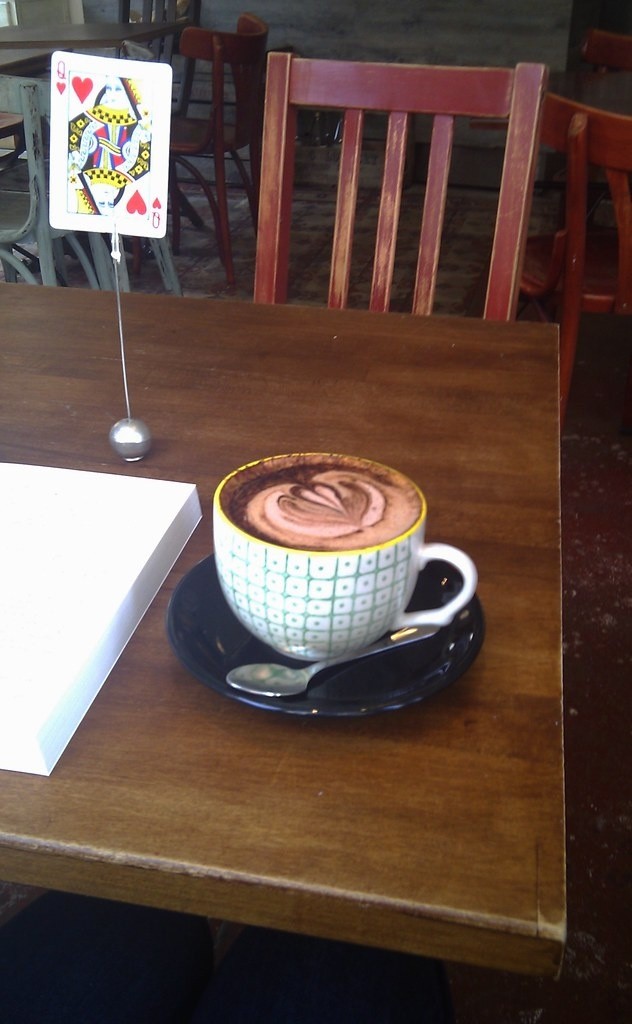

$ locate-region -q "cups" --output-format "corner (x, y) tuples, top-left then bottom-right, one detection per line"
(212, 452), (478, 661)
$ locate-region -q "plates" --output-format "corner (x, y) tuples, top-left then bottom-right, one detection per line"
(166, 553), (484, 716)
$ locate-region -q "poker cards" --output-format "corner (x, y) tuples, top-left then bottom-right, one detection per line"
(45, 48), (174, 240)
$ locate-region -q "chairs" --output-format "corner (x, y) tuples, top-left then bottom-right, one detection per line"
(253, 50), (548, 323)
(0, 72), (185, 296)
(515, 27), (632, 425)
(124, 15), (272, 290)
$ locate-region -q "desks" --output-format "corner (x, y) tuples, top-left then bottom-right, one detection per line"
(1, 20), (195, 291)
(549, 70), (632, 114)
(1, 280), (567, 1024)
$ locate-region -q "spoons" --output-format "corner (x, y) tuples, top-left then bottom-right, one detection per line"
(227, 624), (442, 697)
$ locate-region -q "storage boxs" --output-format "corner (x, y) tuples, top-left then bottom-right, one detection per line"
(1, 461), (203, 777)
